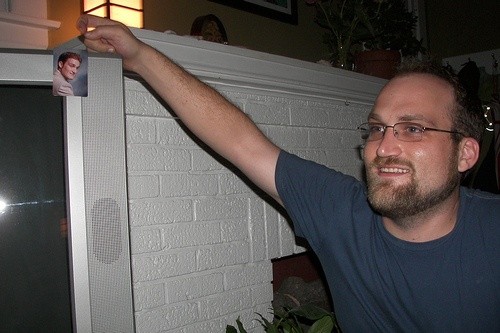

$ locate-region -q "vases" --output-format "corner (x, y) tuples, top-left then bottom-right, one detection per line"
(329, 40), (352, 71)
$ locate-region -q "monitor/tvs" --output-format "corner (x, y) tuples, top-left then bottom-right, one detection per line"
(0, 47), (137, 333)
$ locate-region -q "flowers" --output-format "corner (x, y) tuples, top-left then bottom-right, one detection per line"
(304, 0), (384, 70)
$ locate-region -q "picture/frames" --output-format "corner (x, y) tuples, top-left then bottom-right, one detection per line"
(208, 0), (299, 26)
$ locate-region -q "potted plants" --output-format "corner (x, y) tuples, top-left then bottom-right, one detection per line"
(353, 0), (419, 80)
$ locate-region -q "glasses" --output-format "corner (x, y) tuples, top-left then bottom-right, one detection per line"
(357, 122), (465, 142)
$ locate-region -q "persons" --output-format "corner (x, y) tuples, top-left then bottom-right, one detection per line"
(77, 13), (500, 333)
(52, 52), (82, 96)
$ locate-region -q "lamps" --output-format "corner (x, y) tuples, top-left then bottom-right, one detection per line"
(80, 0), (145, 31)
